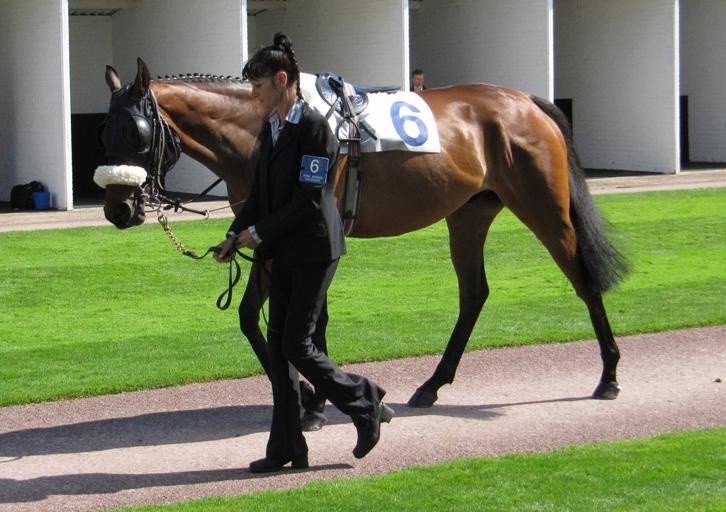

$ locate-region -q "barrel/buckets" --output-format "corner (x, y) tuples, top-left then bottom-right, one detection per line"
(33, 191), (51, 209)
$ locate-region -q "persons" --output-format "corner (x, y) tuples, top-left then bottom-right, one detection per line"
(409, 67), (426, 95)
(213, 28), (395, 472)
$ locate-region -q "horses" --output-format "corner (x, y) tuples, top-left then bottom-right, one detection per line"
(101, 56), (637, 432)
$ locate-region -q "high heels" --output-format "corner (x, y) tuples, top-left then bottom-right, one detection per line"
(250, 450), (309, 472)
(354, 403), (394, 460)
